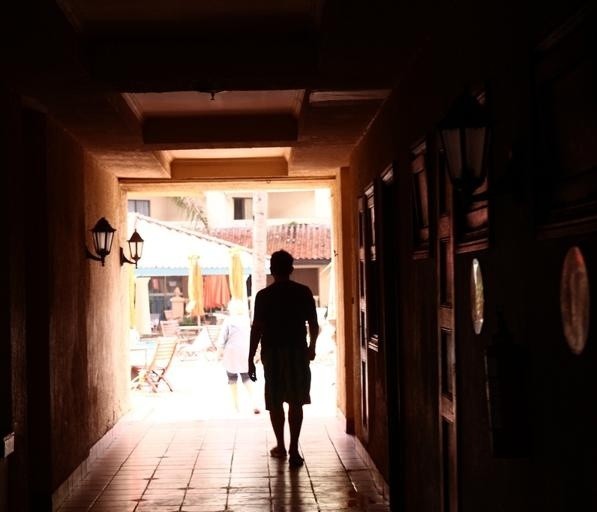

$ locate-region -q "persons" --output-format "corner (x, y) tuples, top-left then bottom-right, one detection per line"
(245, 249), (320, 469)
(215, 296), (252, 382)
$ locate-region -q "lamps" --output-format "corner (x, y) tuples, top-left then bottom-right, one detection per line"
(82, 217), (117, 267)
(433, 84), (569, 244)
(120, 229), (144, 269)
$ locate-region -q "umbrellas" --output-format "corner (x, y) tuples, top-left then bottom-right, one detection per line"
(228, 254), (245, 300)
(186, 258), (205, 332)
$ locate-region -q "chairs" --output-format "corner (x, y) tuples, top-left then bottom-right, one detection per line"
(130, 307), (336, 396)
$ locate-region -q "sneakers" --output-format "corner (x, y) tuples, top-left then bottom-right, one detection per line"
(271, 447), (287, 460)
(288, 451), (304, 465)
